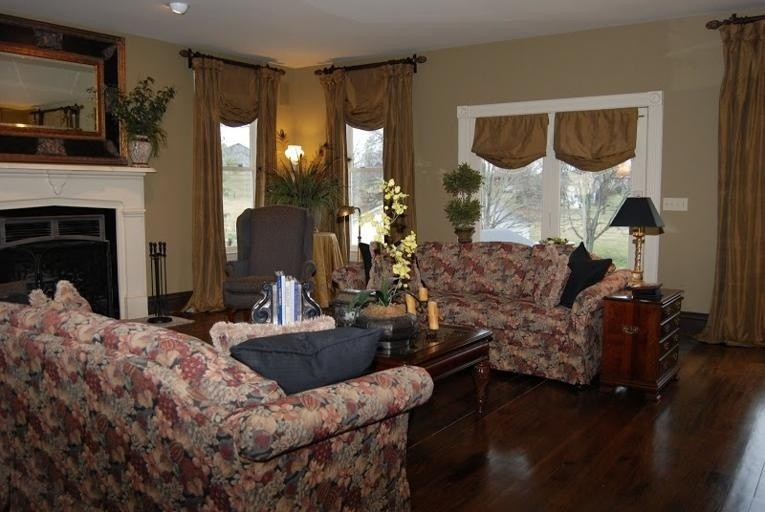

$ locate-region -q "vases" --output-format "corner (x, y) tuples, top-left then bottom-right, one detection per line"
(355, 312), (420, 352)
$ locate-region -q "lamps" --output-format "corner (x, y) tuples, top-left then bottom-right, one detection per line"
(166, 1), (189, 16)
(336, 204), (364, 267)
(608, 195), (667, 290)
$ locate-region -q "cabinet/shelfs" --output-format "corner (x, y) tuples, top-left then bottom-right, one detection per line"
(597, 285), (685, 407)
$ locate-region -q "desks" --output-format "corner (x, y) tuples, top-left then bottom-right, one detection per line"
(311, 230), (349, 311)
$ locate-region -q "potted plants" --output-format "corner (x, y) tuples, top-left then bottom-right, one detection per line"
(441, 160), (485, 242)
(97, 77), (177, 167)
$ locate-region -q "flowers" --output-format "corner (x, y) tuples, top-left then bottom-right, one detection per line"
(350, 178), (418, 312)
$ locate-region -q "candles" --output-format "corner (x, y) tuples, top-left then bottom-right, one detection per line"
(417, 287), (429, 300)
(427, 301), (439, 330)
(405, 293), (418, 315)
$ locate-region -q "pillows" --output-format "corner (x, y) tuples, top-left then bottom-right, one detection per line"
(227, 325), (383, 396)
(208, 313), (337, 356)
(354, 230), (613, 312)
(53, 278), (96, 315)
(26, 287), (52, 307)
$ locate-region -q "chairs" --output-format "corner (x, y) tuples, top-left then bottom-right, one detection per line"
(222, 205), (318, 322)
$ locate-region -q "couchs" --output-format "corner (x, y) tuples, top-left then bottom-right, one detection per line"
(0, 301), (437, 511)
(330, 240), (632, 393)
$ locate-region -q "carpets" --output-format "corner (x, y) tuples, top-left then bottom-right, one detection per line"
(118, 311), (197, 329)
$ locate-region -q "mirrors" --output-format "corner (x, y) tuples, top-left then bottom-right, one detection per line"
(1, 12), (127, 167)
